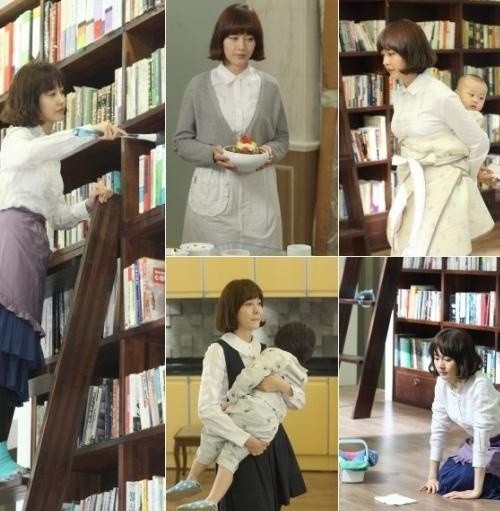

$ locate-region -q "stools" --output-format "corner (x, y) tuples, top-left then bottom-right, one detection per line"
(174, 427), (202, 484)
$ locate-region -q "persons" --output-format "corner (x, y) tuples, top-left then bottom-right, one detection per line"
(1, 63), (129, 490)
(165, 322), (315, 511)
(418, 327), (500, 499)
(165, 278), (309, 510)
(375, 19), (495, 256)
(173, 3), (288, 257)
(454, 75), (489, 125)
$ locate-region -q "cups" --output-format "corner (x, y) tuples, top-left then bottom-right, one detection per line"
(223, 249), (250, 256)
(166, 247), (188, 257)
(179, 242), (214, 255)
(288, 244), (312, 256)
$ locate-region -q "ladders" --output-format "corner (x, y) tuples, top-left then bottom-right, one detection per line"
(1, 188), (122, 511)
(337, 256), (403, 419)
(337, 78), (372, 256)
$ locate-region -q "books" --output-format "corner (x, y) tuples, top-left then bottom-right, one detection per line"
(49, 0), (166, 507)
(487, 67), (500, 98)
(338, 18), (390, 219)
(462, 65), (484, 79)
(0, 0), (49, 100)
(38, 283), (51, 359)
(394, 256), (499, 383)
(460, 20), (499, 50)
(10, 377), (51, 473)
(416, 20), (456, 50)
(427, 66), (452, 88)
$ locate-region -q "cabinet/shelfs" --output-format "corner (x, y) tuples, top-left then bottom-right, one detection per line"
(254, 258), (337, 299)
(339, 0), (500, 253)
(166, 376), (217, 470)
(164, 257), (254, 299)
(0, 1), (164, 511)
(281, 377), (337, 471)
(393, 257), (500, 412)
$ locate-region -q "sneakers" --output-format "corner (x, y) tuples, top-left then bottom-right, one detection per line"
(177, 500), (218, 511)
(0, 442), (29, 482)
(166, 480), (201, 501)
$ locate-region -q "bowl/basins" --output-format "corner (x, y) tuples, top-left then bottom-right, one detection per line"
(223, 145), (267, 171)
